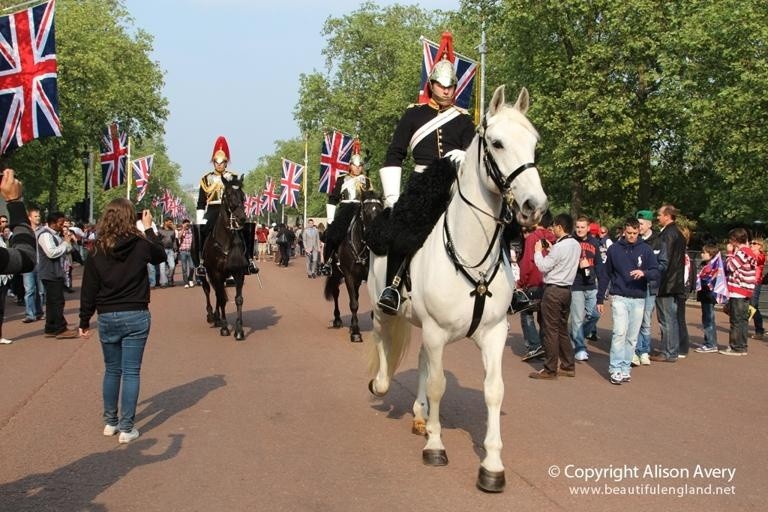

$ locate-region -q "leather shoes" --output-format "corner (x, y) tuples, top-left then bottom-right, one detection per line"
(650, 354), (676, 362)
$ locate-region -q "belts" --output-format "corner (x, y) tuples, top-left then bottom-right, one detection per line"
(545, 284), (570, 289)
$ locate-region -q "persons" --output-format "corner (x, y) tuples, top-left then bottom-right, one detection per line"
(78, 198), (168, 445)
(519, 205), (768, 386)
(0, 150), (326, 347)
(321, 152), (374, 275)
(380, 59), (544, 316)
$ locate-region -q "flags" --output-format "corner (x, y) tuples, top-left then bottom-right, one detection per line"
(317, 126), (356, 196)
(99, 120), (128, 191)
(418, 41), (478, 110)
(0, 0), (64, 159)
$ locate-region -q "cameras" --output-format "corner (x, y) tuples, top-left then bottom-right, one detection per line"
(540, 238), (550, 249)
(136, 212), (146, 220)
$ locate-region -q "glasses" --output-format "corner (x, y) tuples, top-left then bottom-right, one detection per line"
(750, 241), (760, 245)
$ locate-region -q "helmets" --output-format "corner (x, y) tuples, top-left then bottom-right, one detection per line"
(429, 60), (458, 88)
(213, 150), (227, 164)
(350, 154), (364, 167)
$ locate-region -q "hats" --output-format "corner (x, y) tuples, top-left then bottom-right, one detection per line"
(636, 210), (656, 221)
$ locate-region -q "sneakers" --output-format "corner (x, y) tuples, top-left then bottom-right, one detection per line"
(720, 346), (748, 355)
(529, 369), (556, 380)
(521, 345), (546, 361)
(557, 368), (575, 377)
(103, 423), (121, 436)
(640, 353), (651, 364)
(752, 330), (768, 339)
(119, 429), (139, 443)
(37, 316), (45, 320)
(45, 333), (56, 337)
(575, 351), (589, 360)
(622, 373), (632, 382)
(56, 330), (78, 338)
(0, 338), (12, 344)
(631, 355), (640, 366)
(189, 281), (194, 287)
(184, 284), (189, 288)
(609, 371), (623, 385)
(695, 346), (718, 353)
(22, 319), (34, 323)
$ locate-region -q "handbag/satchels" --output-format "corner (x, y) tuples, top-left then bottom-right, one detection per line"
(723, 302), (756, 324)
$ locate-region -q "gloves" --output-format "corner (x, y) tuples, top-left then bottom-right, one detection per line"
(326, 204), (336, 225)
(379, 166), (402, 210)
(444, 149), (466, 164)
(196, 210), (208, 224)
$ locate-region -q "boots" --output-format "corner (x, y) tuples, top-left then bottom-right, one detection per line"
(244, 223), (259, 273)
(377, 252), (409, 309)
(193, 224), (208, 274)
(322, 230), (338, 275)
(508, 289), (530, 311)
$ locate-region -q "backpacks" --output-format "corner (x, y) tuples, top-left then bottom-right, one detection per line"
(276, 230), (289, 244)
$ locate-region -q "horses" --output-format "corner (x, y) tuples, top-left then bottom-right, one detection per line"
(324, 186), (384, 342)
(367, 84), (549, 492)
(201, 173), (247, 340)
